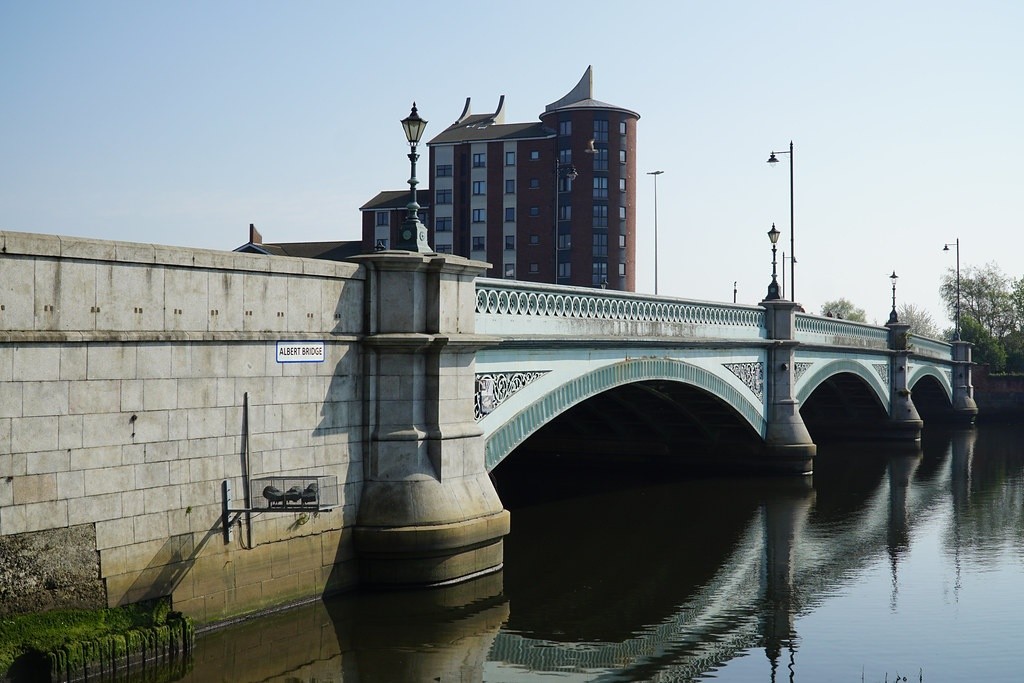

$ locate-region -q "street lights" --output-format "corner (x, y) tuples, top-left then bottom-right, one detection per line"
(765, 222), (783, 299)
(887, 270), (899, 323)
(554, 163), (578, 284)
(396, 101), (433, 253)
(647, 171), (665, 295)
(734, 281), (737, 304)
(767, 150), (794, 302)
(943, 243), (960, 341)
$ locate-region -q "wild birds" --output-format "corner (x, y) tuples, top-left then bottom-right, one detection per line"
(263, 482), (320, 509)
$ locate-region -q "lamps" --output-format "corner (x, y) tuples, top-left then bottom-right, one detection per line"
(263, 483), (321, 509)
